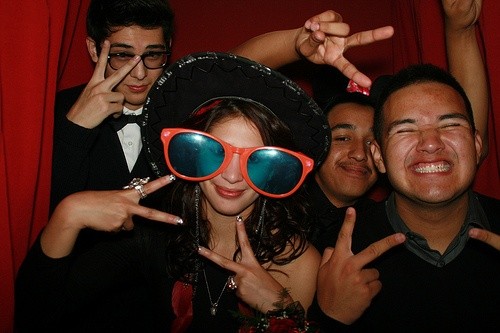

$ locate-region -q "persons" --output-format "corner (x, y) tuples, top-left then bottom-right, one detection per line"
(48, 0), (178, 269)
(232, 0), (492, 269)
(16, 49), (332, 332)
(299, 62), (500, 332)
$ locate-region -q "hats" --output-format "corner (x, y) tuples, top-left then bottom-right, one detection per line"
(140, 51), (332, 191)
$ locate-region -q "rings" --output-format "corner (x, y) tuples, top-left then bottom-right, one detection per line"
(227, 276), (238, 290)
(123, 177), (151, 200)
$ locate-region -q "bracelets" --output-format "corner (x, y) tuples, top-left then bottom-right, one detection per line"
(228, 286), (315, 333)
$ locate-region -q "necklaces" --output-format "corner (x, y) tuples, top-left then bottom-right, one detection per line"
(201, 256), (231, 318)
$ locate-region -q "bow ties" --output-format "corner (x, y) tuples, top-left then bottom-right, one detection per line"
(113, 111), (143, 131)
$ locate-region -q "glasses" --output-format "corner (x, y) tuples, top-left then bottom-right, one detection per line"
(158, 127), (314, 198)
(95, 45), (170, 70)
